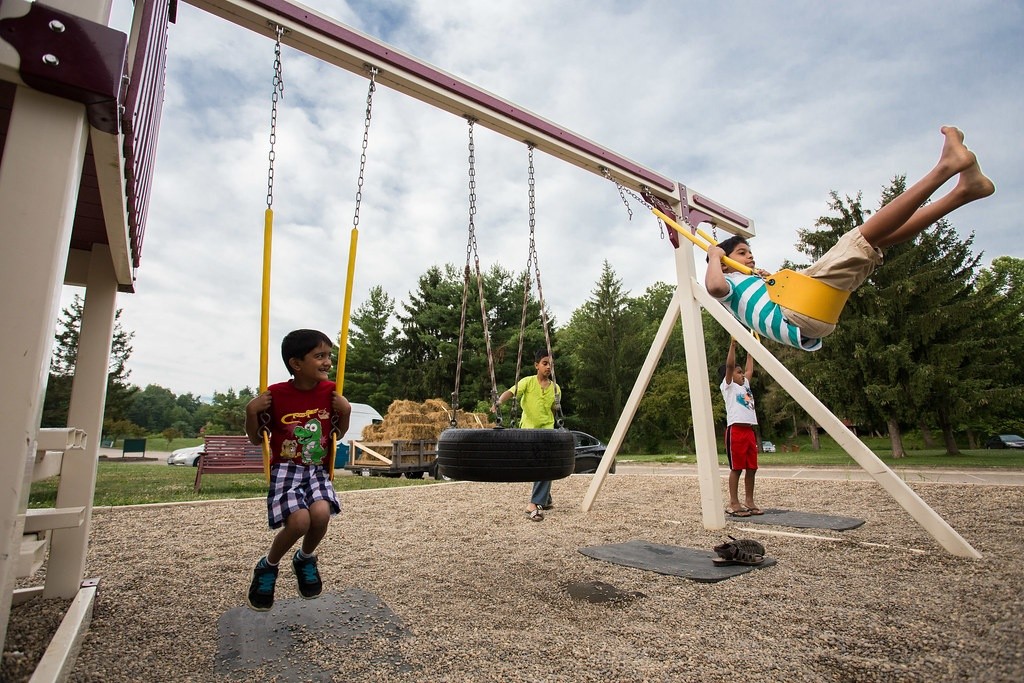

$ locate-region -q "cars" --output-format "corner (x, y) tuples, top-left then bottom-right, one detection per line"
(166, 439), (253, 467)
(986, 434), (1024, 449)
(762, 441), (776, 453)
(571, 430), (616, 475)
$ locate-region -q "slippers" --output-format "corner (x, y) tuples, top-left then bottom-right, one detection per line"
(750, 507), (764, 515)
(536, 504), (552, 510)
(725, 507), (751, 517)
(528, 510), (544, 520)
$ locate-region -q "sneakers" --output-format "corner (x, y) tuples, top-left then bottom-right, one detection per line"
(290, 549), (323, 600)
(246, 556), (279, 612)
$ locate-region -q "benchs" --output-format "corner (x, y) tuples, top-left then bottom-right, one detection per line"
(123, 438), (146, 458)
(194, 436), (264, 492)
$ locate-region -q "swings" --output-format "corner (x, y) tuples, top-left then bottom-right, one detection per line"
(259, 19), (376, 487)
(438, 121), (575, 484)
(598, 171), (850, 327)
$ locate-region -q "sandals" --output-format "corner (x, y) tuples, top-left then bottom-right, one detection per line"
(712, 543), (764, 565)
(727, 536), (765, 556)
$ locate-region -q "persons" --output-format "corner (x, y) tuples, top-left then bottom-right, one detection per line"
(720, 335), (764, 516)
(493, 348), (561, 521)
(244, 329), (351, 612)
(708, 127), (995, 349)
